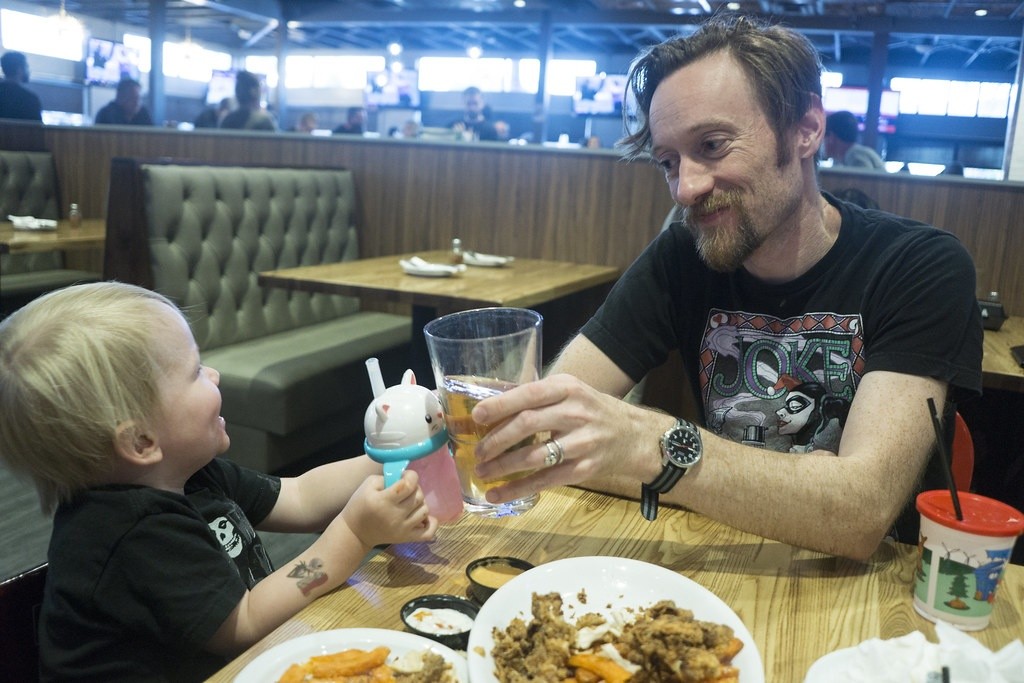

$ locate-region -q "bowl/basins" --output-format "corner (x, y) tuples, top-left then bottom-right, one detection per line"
(466, 557), (535, 604)
(466, 556), (763, 683)
(400, 593), (481, 650)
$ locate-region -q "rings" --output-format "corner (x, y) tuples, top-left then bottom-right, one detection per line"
(543, 437), (565, 467)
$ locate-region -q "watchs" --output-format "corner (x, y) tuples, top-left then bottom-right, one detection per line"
(640, 416), (702, 521)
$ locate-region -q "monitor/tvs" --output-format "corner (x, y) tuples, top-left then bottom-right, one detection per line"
(367, 70), (421, 107)
(205, 70), (267, 106)
(573, 76), (638, 122)
(83, 37), (140, 88)
(824, 87), (900, 135)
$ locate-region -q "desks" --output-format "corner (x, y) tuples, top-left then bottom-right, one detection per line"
(256, 250), (623, 381)
(0, 218), (107, 255)
(981, 313), (1024, 388)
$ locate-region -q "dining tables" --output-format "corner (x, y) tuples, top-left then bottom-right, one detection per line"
(202, 485), (1024, 683)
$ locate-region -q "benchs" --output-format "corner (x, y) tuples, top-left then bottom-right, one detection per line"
(0, 151), (103, 299)
(97, 158), (412, 475)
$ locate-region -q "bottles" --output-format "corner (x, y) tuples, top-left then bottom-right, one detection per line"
(452, 238), (462, 263)
(69, 204), (82, 229)
(360, 358), (464, 529)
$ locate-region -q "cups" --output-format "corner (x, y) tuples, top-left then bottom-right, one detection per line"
(805, 642), (989, 682)
(423, 306), (544, 519)
(912, 489), (1024, 631)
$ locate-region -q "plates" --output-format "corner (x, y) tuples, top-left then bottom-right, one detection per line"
(13, 219), (57, 231)
(402, 262), (466, 276)
(233, 627), (467, 683)
(463, 250), (515, 267)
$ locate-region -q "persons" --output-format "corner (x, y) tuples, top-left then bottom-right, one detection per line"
(194, 97), (237, 128)
(0, 283), (438, 683)
(333, 105), (368, 134)
(222, 72), (281, 131)
(290, 111), (321, 132)
(448, 84), (498, 141)
(96, 79), (153, 127)
(464, 8), (987, 562)
(0, 52), (43, 122)
(823, 111), (887, 172)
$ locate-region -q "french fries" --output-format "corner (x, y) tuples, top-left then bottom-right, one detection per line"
(277, 646), (397, 683)
(569, 653), (634, 683)
